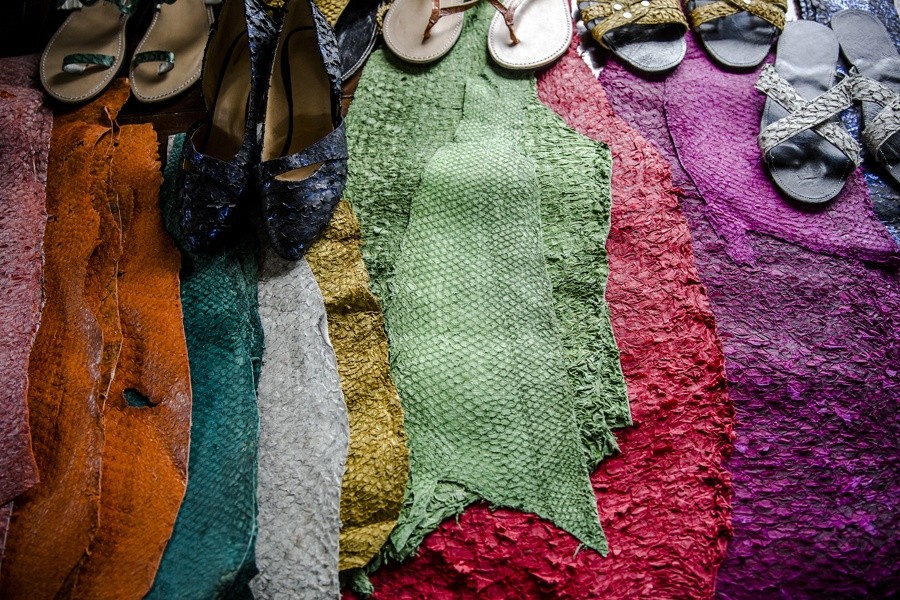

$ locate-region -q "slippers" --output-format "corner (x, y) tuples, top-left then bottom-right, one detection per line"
(487, 1), (574, 70)
(691, 0), (788, 68)
(577, 0), (689, 73)
(382, 0), (482, 63)
(40, 0), (132, 102)
(128, 0), (225, 103)
(831, 9), (900, 183)
(753, 20), (863, 203)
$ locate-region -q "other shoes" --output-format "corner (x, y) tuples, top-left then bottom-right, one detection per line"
(256, 1), (349, 259)
(162, 1), (278, 253)
